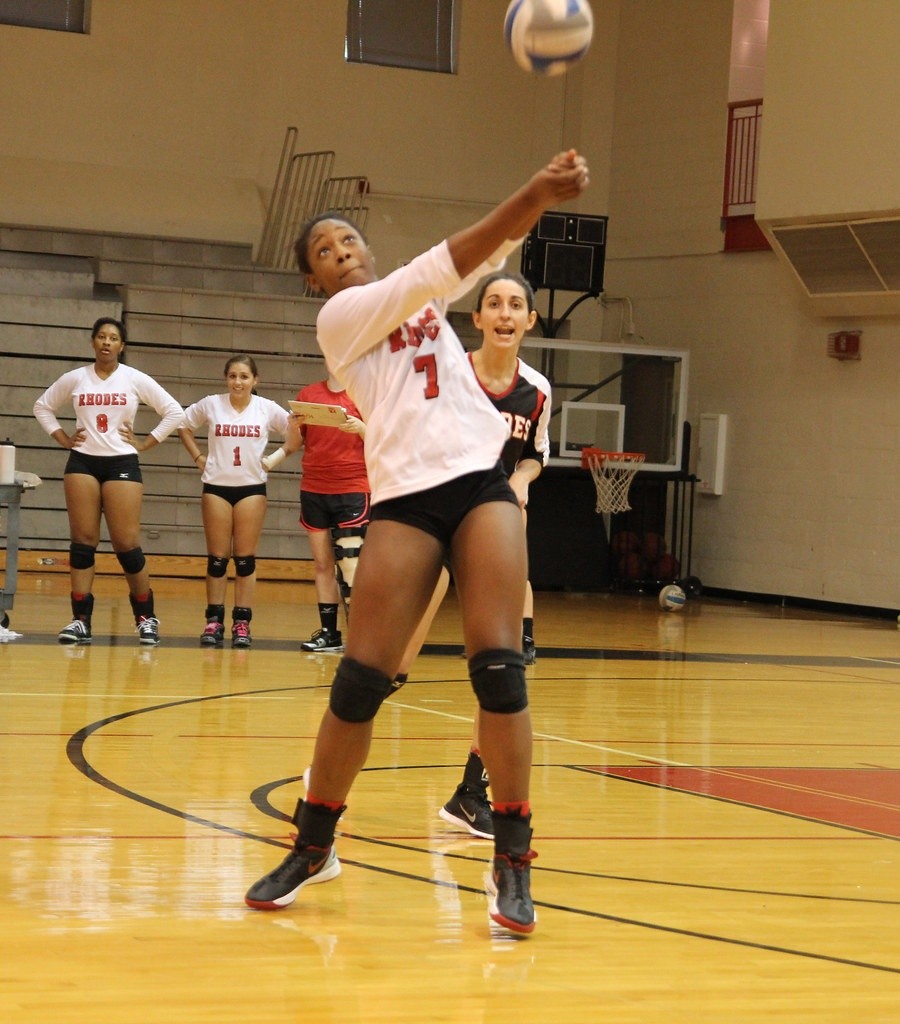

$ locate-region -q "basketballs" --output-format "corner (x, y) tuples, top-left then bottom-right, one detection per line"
(611, 531), (681, 579)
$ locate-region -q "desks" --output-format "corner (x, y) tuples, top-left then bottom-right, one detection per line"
(0, 481), (36, 629)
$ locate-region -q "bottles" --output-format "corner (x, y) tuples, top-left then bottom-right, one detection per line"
(0, 437), (16, 484)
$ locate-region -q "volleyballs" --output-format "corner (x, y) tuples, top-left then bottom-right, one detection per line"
(504, 0), (593, 78)
(658, 585), (687, 611)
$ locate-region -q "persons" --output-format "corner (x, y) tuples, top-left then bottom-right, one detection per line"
(303, 274), (552, 839)
(176, 355), (303, 646)
(33, 317), (185, 644)
(285, 360), (371, 652)
(244, 149), (590, 934)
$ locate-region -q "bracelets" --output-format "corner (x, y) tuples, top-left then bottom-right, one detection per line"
(195, 454), (202, 462)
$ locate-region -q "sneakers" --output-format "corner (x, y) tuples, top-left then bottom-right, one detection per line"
(301, 627), (343, 653)
(200, 616), (224, 644)
(482, 850), (539, 934)
(57, 620), (92, 643)
(439, 782), (495, 839)
(135, 614), (160, 644)
(522, 643), (536, 666)
(231, 618), (252, 646)
(244, 831), (343, 909)
